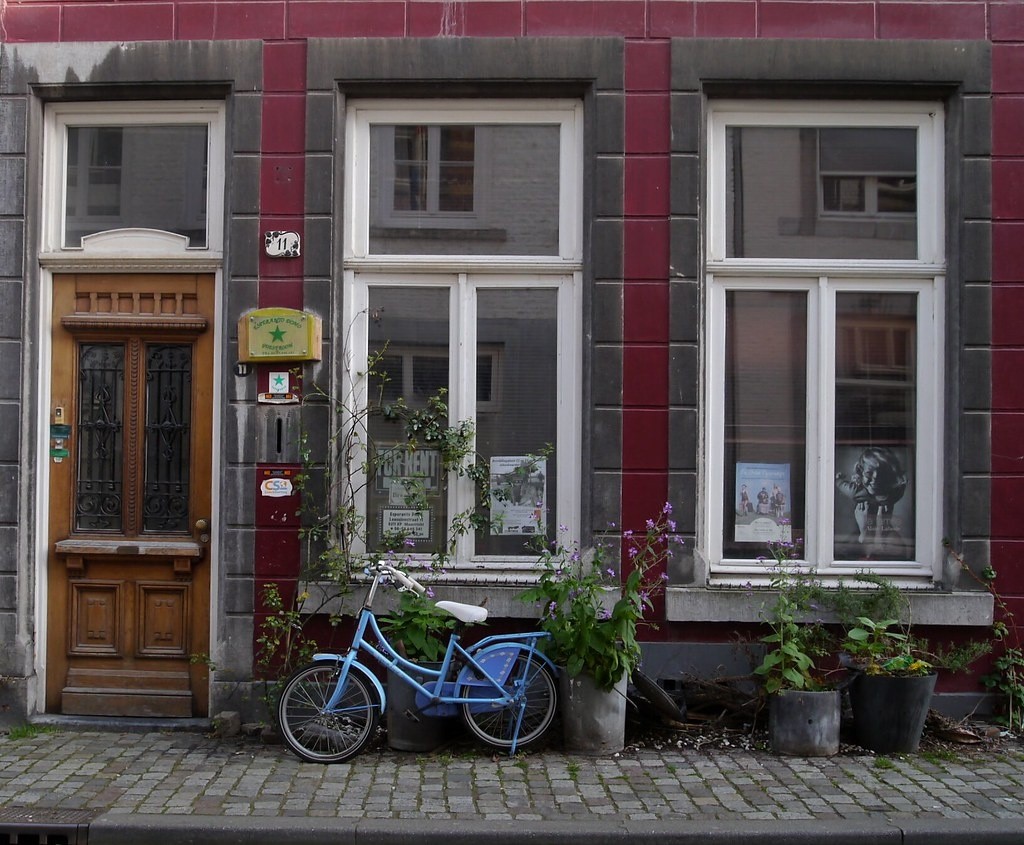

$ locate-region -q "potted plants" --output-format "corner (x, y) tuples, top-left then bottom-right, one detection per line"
(839, 616), (939, 752)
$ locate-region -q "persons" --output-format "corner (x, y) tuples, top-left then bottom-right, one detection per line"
(740, 484), (785, 518)
(836, 446), (907, 561)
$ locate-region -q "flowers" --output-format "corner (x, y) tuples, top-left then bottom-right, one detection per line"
(744, 516), (833, 696)
(515, 495), (688, 709)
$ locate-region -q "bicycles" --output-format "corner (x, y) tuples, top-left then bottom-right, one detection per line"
(276, 565), (564, 765)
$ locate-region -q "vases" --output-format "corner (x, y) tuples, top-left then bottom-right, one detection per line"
(770, 690), (841, 760)
(551, 663), (626, 755)
